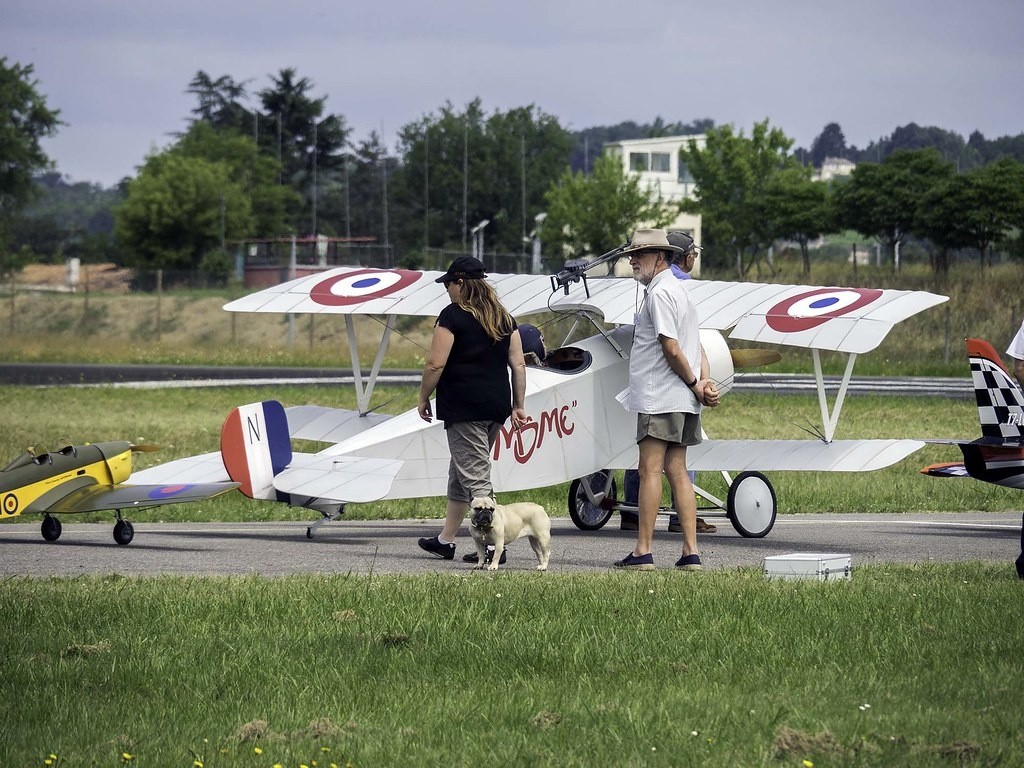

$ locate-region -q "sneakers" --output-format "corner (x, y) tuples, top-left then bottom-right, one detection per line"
(673, 554), (701, 571)
(618, 511), (639, 530)
(669, 516), (717, 533)
(462, 549), (507, 564)
(418, 536), (456, 561)
(614, 551), (656, 572)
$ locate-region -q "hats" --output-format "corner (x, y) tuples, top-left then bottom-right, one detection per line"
(618, 229), (684, 255)
(666, 231), (705, 253)
(518, 324), (547, 367)
(434, 256), (487, 283)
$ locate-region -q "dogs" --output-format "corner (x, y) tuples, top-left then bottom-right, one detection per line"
(468, 487), (552, 572)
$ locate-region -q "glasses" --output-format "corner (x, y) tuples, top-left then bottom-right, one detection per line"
(626, 250), (658, 260)
(444, 282), (458, 288)
(685, 251), (698, 258)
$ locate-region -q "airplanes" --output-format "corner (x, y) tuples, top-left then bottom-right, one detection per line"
(0, 441), (244, 546)
(120, 264), (949, 539)
(920, 337), (1024, 491)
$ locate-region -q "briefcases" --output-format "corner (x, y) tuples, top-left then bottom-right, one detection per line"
(762, 554), (852, 586)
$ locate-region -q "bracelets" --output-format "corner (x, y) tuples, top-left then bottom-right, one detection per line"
(687, 375), (697, 387)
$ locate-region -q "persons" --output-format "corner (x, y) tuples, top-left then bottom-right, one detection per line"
(612, 228), (720, 571)
(621, 231), (717, 533)
(418, 255), (526, 563)
(1006, 322), (1024, 580)
